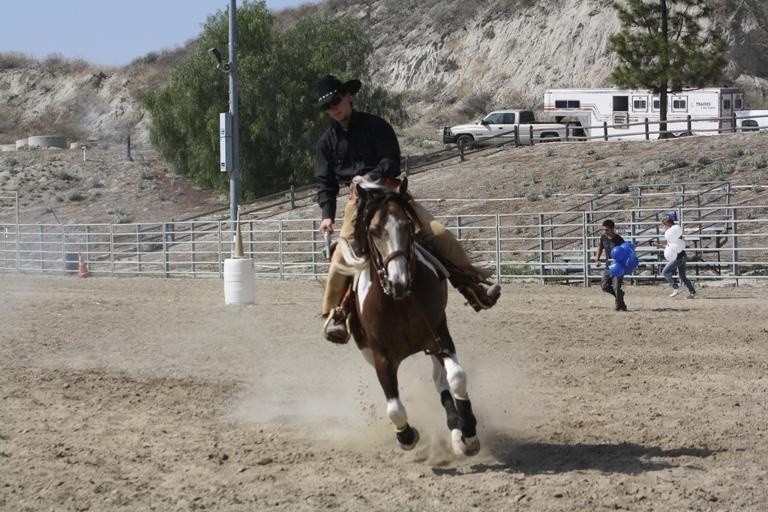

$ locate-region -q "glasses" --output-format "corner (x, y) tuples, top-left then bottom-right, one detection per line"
(322, 91), (347, 110)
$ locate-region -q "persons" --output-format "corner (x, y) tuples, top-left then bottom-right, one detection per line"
(310, 74), (502, 342)
(594, 219), (627, 312)
(652, 217), (697, 298)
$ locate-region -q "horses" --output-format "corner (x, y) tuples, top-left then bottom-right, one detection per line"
(341, 174), (483, 458)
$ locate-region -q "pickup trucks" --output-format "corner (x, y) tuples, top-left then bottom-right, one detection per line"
(443, 109), (584, 147)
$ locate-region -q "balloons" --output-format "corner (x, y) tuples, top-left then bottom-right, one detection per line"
(663, 225), (685, 262)
(608, 242), (639, 277)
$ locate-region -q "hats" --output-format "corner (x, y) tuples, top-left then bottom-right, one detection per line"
(309, 74), (362, 109)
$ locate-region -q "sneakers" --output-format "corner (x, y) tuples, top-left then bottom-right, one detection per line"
(459, 280), (501, 313)
(669, 288), (698, 299)
(326, 312), (348, 344)
